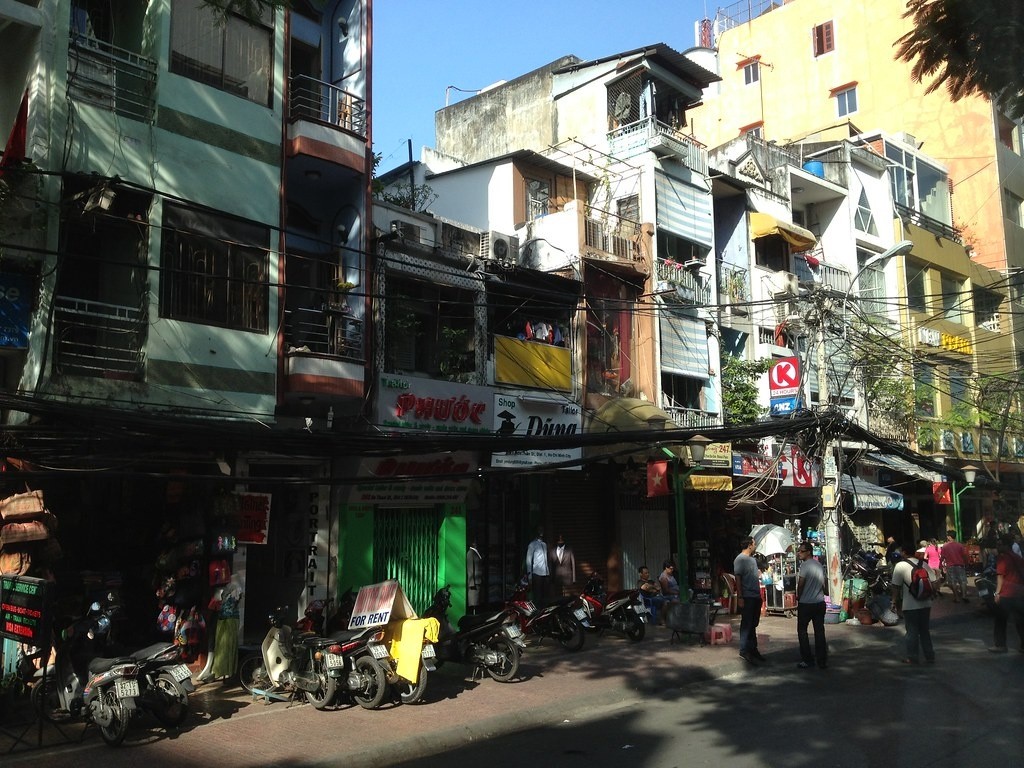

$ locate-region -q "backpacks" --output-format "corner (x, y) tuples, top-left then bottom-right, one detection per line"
(904, 558), (933, 601)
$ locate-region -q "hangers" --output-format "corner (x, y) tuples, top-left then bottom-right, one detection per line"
(519, 314), (567, 326)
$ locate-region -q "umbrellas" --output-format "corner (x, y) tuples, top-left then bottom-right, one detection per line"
(749, 524), (794, 557)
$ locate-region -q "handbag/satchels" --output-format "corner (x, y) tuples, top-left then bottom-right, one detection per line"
(0, 475), (242, 664)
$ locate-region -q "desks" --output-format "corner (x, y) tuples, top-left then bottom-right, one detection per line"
(709, 606), (722, 626)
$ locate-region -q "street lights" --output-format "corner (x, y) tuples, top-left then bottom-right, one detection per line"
(814, 238), (915, 611)
(951, 464), (979, 543)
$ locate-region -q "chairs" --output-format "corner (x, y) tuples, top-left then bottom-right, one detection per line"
(640, 596), (673, 625)
(723, 574), (742, 617)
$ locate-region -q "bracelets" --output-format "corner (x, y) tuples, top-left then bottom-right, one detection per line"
(890, 601), (896, 604)
(995, 593), (999, 595)
(738, 596), (743, 599)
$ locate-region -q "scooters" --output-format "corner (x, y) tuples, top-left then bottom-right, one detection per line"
(236, 586), (439, 710)
(972, 556), (1000, 617)
(845, 541), (902, 604)
(30, 591), (195, 748)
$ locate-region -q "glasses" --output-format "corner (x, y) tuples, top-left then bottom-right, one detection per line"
(797, 548), (809, 552)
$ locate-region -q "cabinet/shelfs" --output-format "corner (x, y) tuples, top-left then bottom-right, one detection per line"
(765, 547), (797, 618)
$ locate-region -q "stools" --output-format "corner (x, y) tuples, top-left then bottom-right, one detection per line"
(714, 623), (732, 641)
(712, 628), (727, 645)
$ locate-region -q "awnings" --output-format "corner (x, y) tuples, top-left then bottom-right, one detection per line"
(749, 212), (818, 253)
(838, 473), (903, 509)
(867, 454), (950, 504)
(584, 397), (688, 464)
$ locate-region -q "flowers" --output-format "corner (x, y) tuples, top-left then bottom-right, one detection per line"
(337, 282), (354, 289)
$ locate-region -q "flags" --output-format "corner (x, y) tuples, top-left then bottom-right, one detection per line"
(644, 462), (670, 498)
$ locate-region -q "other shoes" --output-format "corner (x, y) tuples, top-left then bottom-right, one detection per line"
(902, 656), (919, 665)
(925, 654), (936, 663)
(797, 658), (815, 669)
(815, 656), (828, 666)
(953, 596), (960, 602)
(987, 644), (1008, 653)
(752, 652), (767, 663)
(1019, 649), (1024, 653)
(961, 596), (969, 603)
(740, 653), (757, 667)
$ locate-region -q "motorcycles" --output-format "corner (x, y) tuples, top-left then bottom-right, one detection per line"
(420, 582), (527, 684)
(577, 568), (652, 642)
(505, 570), (586, 651)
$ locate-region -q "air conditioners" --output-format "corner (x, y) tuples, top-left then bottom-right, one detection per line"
(481, 231), (519, 265)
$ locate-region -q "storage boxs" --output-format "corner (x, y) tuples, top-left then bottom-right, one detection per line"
(824, 612), (840, 624)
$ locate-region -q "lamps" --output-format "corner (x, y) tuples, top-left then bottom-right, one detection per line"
(338, 17), (347, 37)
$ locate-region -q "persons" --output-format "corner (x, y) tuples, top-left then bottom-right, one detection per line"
(467, 541), (485, 606)
(637, 567), (677, 625)
(890, 542), (936, 664)
(733, 536), (765, 666)
(793, 543), (828, 669)
(526, 526), (549, 593)
(659, 561), (693, 600)
(549, 541), (575, 591)
(987, 535), (1024, 651)
(885, 530), (969, 602)
(976, 509), (1024, 570)
